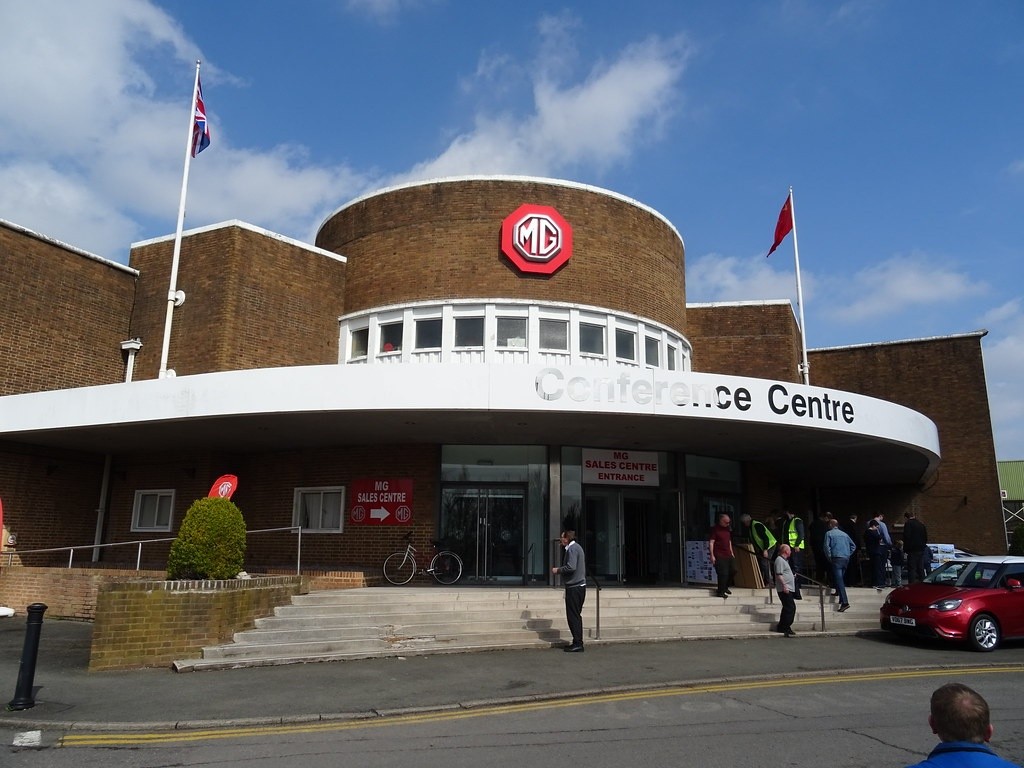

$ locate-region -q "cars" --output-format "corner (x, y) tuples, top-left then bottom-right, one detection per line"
(878, 555), (1024, 653)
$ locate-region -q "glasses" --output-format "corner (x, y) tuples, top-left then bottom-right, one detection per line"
(560, 536), (565, 539)
(724, 521), (730, 524)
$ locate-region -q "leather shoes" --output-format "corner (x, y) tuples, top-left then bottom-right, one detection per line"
(564, 644), (584, 652)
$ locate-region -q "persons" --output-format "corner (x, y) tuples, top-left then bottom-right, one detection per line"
(551, 529), (588, 652)
(773, 542), (798, 635)
(907, 682), (1021, 767)
(382, 338), (395, 351)
(707, 505), (934, 611)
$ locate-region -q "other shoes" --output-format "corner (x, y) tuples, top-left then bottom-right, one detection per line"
(831, 592), (839, 596)
(777, 624), (796, 635)
(725, 589), (731, 594)
(891, 584), (902, 588)
(717, 592), (728, 598)
(765, 581), (775, 589)
(872, 584), (885, 589)
(837, 604), (850, 612)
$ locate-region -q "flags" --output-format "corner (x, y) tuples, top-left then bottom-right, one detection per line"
(766, 192), (792, 257)
(191, 74), (210, 157)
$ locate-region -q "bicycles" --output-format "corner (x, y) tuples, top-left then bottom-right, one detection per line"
(382, 530), (463, 585)
